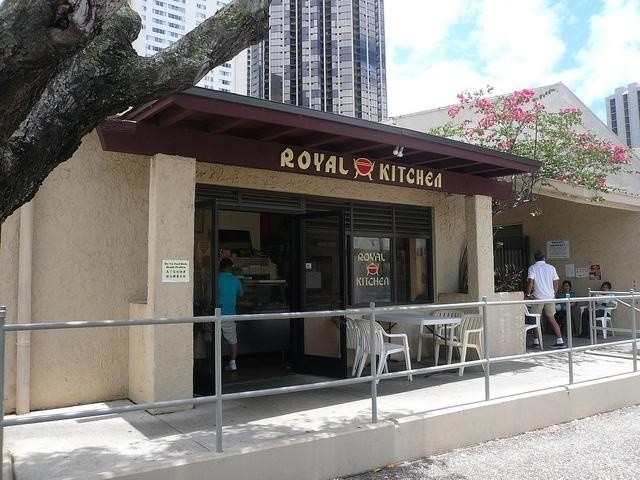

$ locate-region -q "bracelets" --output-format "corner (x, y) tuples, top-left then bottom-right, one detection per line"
(525, 293), (530, 297)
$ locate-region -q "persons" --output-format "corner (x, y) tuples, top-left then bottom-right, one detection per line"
(556, 280), (578, 336)
(576, 282), (616, 339)
(205, 257), (245, 372)
(524, 249), (566, 349)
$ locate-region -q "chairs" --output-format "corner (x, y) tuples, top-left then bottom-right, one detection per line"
(579, 294), (617, 339)
(346, 309), (484, 385)
(524, 304), (545, 351)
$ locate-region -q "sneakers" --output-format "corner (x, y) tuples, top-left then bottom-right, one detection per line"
(528, 342), (541, 349)
(550, 342), (566, 349)
(578, 332), (599, 338)
(224, 359), (237, 371)
